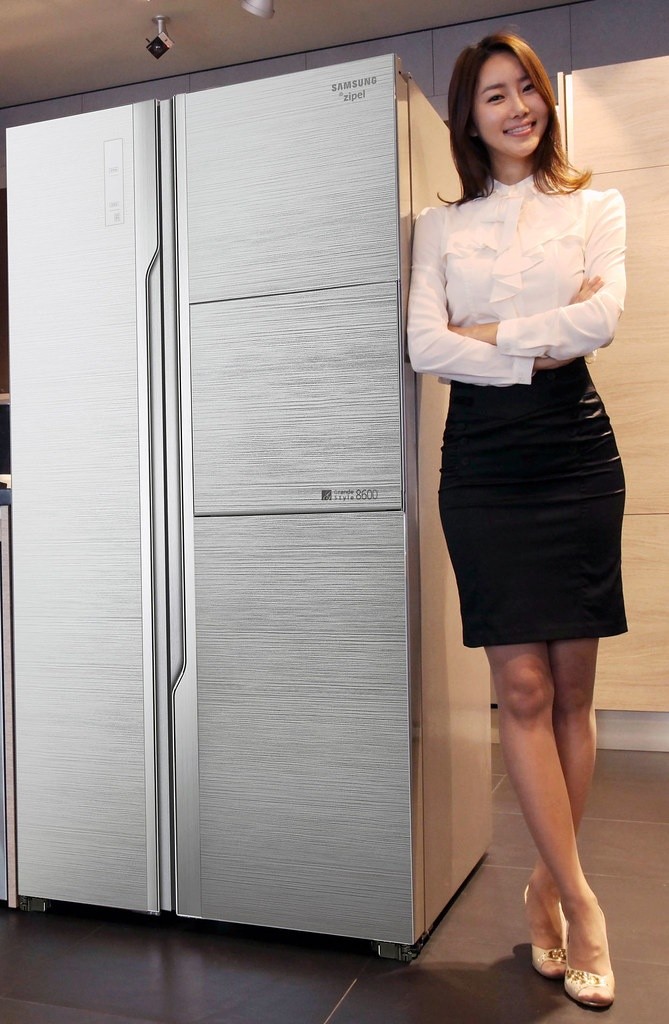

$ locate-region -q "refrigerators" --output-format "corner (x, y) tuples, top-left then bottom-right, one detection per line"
(7, 55), (494, 944)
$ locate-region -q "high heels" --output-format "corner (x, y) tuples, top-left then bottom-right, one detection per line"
(524, 884), (569, 980)
(564, 905), (615, 1007)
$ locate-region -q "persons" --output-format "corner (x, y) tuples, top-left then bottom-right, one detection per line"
(406, 33), (629, 1007)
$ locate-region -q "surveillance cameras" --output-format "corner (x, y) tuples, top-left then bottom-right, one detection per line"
(146, 32), (173, 60)
